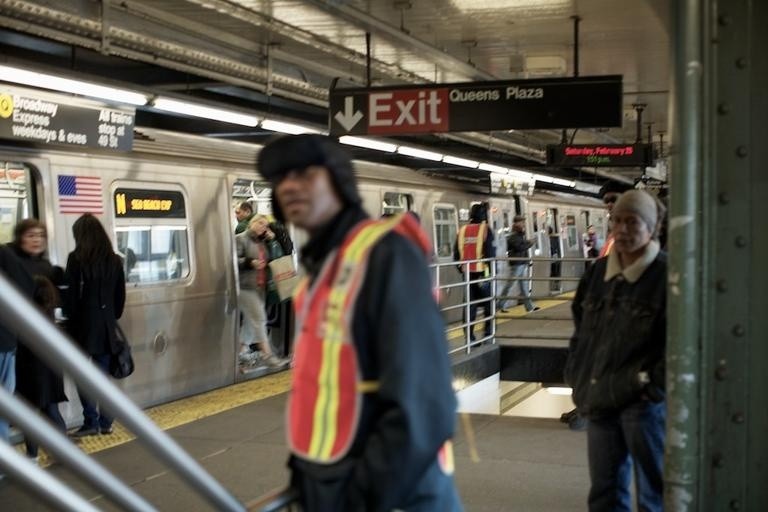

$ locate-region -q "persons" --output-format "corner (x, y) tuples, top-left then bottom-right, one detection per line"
(0, 213), (126, 463)
(235, 201), (293, 374)
(250, 131), (474, 512)
(497, 215), (540, 313)
(598, 179), (637, 259)
(453, 205), (497, 347)
(582, 227), (597, 258)
(567, 188), (668, 511)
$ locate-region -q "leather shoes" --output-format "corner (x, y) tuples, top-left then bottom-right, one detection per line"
(560, 408), (578, 422)
(69, 426), (113, 437)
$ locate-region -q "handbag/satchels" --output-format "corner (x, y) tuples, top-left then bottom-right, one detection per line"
(268, 255), (298, 302)
(109, 340), (134, 379)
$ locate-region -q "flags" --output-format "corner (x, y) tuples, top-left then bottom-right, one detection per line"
(57, 172), (104, 216)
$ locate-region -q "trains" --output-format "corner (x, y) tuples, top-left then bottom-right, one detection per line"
(1, 147), (610, 447)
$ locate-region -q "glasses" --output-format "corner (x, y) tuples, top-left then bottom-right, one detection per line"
(604, 195), (617, 204)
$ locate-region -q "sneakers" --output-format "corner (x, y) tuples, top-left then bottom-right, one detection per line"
(238, 351), (258, 367)
(259, 352), (290, 369)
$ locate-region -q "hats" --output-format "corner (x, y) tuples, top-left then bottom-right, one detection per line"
(470, 202), (489, 218)
(611, 189), (658, 235)
(599, 181), (634, 199)
(513, 215), (527, 222)
(256, 133), (362, 226)
